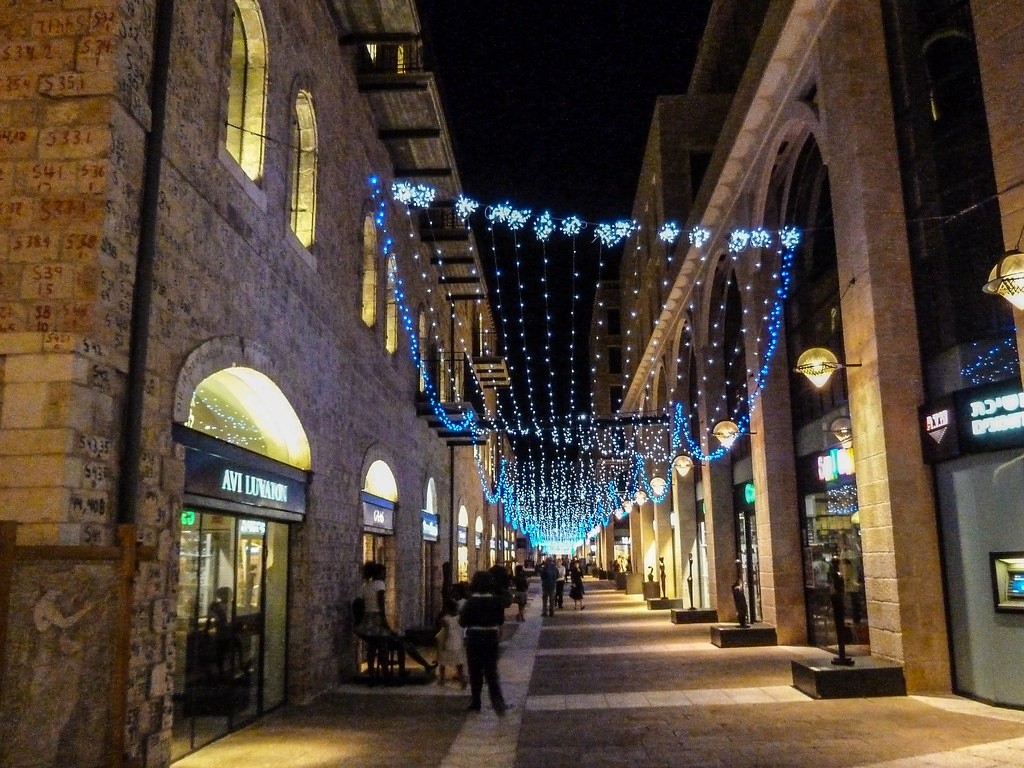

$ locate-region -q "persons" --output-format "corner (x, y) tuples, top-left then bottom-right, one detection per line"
(437, 565), (514, 714)
(611, 561), (621, 579)
(360, 563), (438, 671)
(216, 586), (235, 623)
(626, 560), (632, 572)
(515, 565), (528, 622)
(541, 556), (586, 616)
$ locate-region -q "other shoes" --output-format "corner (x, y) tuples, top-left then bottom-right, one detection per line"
(574, 606), (578, 609)
(398, 669), (411, 677)
(521, 617), (525, 622)
(581, 605), (586, 610)
(426, 660), (439, 672)
(541, 613), (546, 616)
(558, 605), (564, 609)
(517, 614), (521, 621)
(554, 604), (558, 607)
(497, 702), (516, 713)
(468, 702), (482, 712)
(548, 614), (554, 617)
